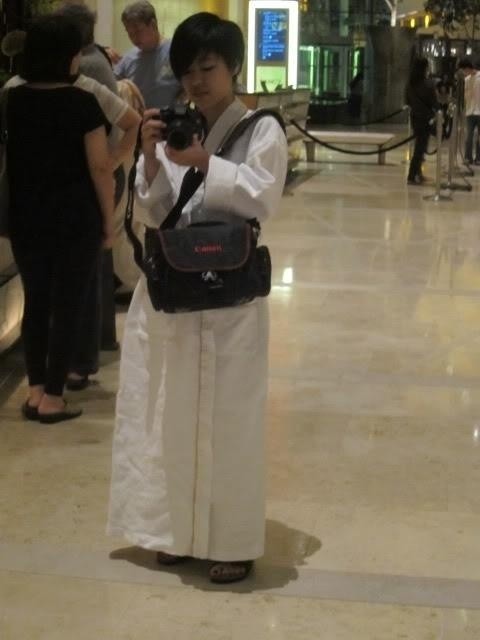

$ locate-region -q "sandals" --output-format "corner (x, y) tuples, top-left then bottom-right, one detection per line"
(156, 551), (252, 584)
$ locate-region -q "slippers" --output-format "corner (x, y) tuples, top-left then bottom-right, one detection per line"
(21, 397), (82, 424)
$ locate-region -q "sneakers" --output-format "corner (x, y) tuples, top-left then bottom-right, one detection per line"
(66, 373), (88, 389)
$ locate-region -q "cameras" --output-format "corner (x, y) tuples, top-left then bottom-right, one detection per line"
(152, 99), (209, 154)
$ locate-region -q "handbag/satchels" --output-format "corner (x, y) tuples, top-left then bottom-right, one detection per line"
(150, 218), (271, 314)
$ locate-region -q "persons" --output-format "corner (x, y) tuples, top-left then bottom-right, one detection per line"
(1, 0), (183, 425)
(104, 9), (291, 585)
(404, 54), (480, 188)
